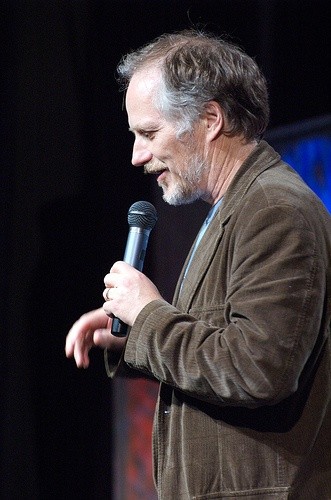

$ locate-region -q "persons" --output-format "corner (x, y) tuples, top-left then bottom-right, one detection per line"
(64, 28), (330, 500)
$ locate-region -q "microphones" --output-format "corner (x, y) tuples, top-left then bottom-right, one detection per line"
(111, 201), (157, 337)
